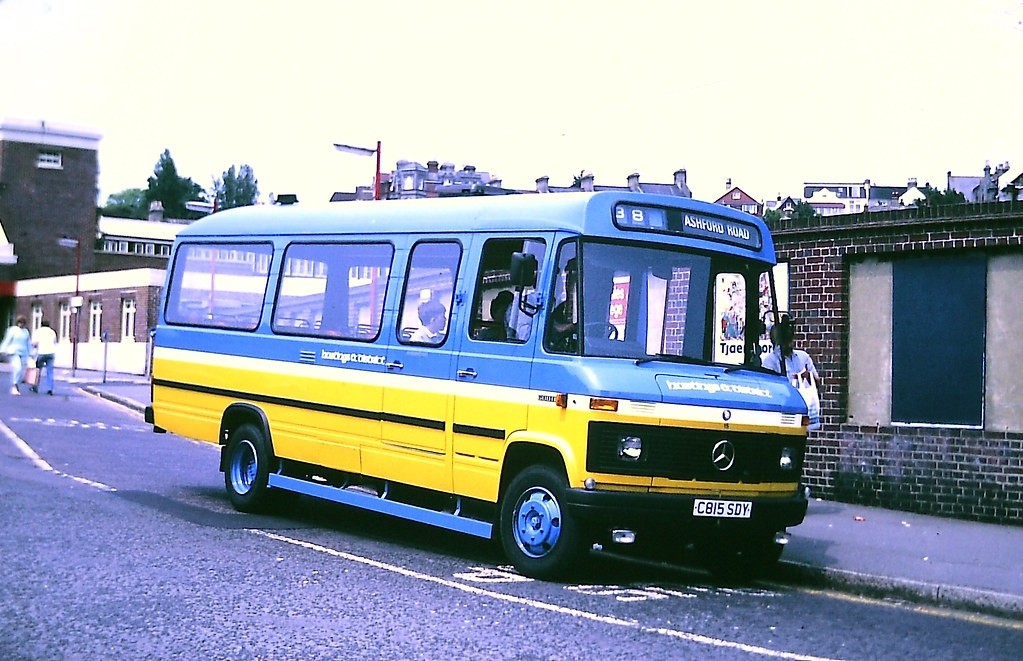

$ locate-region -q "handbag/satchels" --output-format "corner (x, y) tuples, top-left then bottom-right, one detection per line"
(23, 367), (40, 384)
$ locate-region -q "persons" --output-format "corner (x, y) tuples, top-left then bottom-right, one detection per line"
(0, 315), (32, 396)
(29, 318), (57, 396)
(407, 273), (579, 354)
(761, 323), (821, 392)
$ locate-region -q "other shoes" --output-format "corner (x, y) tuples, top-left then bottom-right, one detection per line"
(28, 385), (39, 393)
(46, 390), (53, 396)
(11, 385), (20, 396)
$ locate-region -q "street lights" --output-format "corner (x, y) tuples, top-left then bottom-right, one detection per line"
(332, 141), (381, 337)
(185, 198), (219, 314)
(59, 239), (82, 377)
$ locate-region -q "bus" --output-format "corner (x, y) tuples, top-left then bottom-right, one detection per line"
(143, 191), (809, 579)
